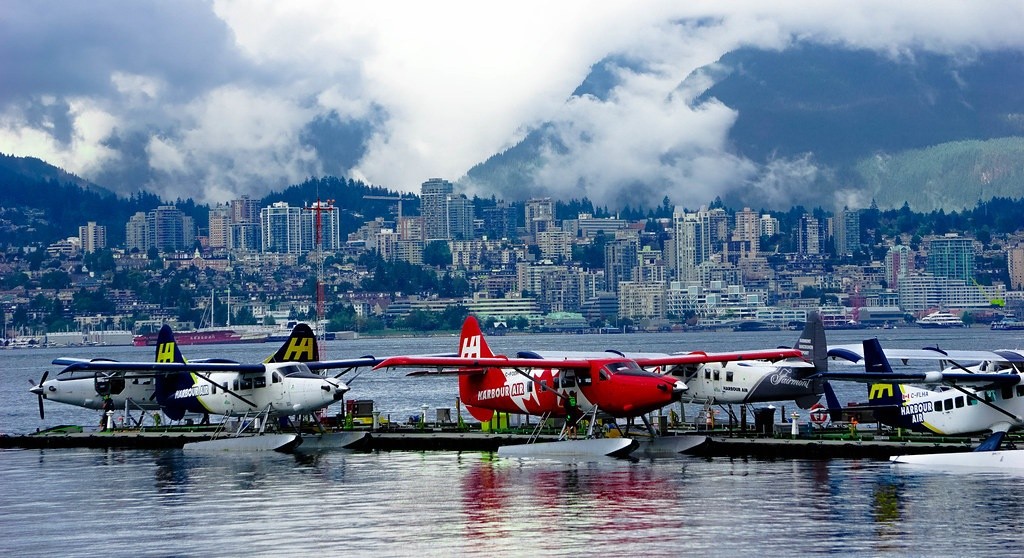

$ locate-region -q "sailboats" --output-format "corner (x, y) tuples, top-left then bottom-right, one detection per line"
(0, 319), (107, 348)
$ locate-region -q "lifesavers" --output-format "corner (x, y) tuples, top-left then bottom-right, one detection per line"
(809, 404), (827, 425)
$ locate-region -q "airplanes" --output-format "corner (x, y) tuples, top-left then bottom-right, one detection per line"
(371, 315), (804, 459)
(642, 309), (1024, 474)
(28, 322), (460, 451)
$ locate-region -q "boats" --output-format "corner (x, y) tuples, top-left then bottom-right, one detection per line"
(915, 309), (964, 329)
(133, 287), (243, 347)
(990, 313), (1024, 330)
